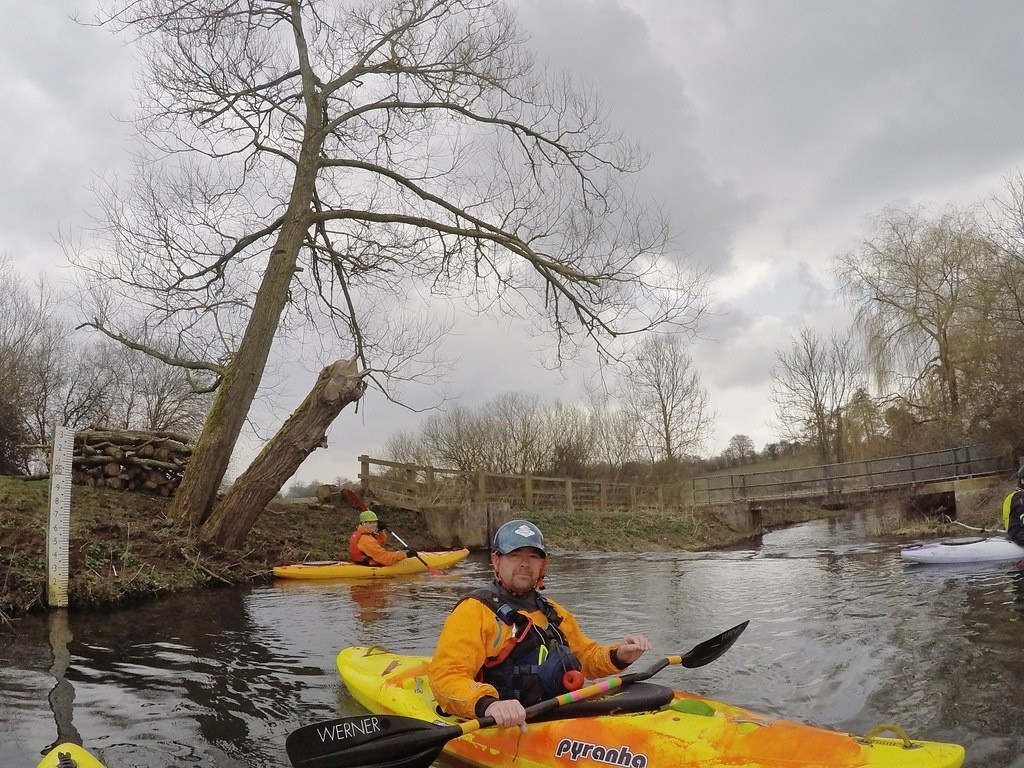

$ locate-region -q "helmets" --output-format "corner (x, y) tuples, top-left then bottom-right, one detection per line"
(359, 511), (378, 523)
(1017, 466), (1024, 479)
(491, 520), (548, 559)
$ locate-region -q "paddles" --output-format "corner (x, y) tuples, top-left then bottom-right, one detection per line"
(283, 617), (753, 768)
(339, 488), (442, 576)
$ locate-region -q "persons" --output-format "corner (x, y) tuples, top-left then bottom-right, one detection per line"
(1002, 466), (1024, 546)
(429, 519), (653, 734)
(352, 511), (417, 566)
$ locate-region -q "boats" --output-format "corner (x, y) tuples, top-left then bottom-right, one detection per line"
(899, 534), (1024, 567)
(336, 644), (967, 768)
(272, 548), (471, 580)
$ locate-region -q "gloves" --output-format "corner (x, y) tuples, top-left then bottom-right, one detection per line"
(377, 520), (385, 532)
(407, 550), (417, 558)
